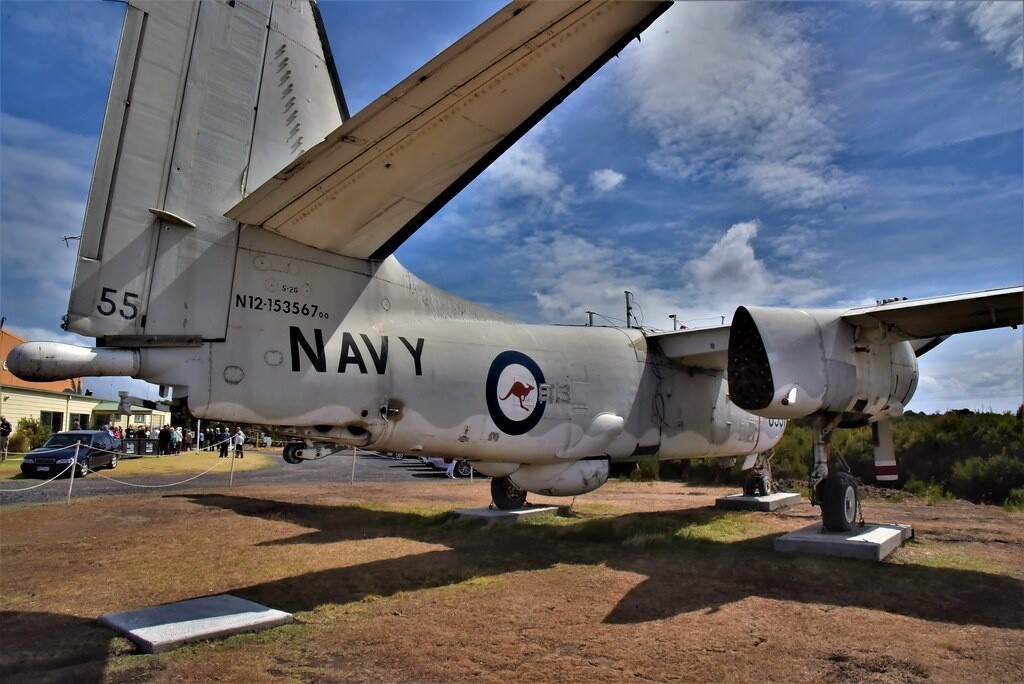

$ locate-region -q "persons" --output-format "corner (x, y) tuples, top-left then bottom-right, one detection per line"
(71, 419), (245, 459)
(0, 415), (13, 463)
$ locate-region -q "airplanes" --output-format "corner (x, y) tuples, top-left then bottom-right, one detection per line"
(4, 1), (1024, 537)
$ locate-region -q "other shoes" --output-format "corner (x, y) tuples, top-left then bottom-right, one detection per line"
(450, 476), (456, 479)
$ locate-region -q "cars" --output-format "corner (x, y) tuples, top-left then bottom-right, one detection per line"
(21, 429), (119, 478)
